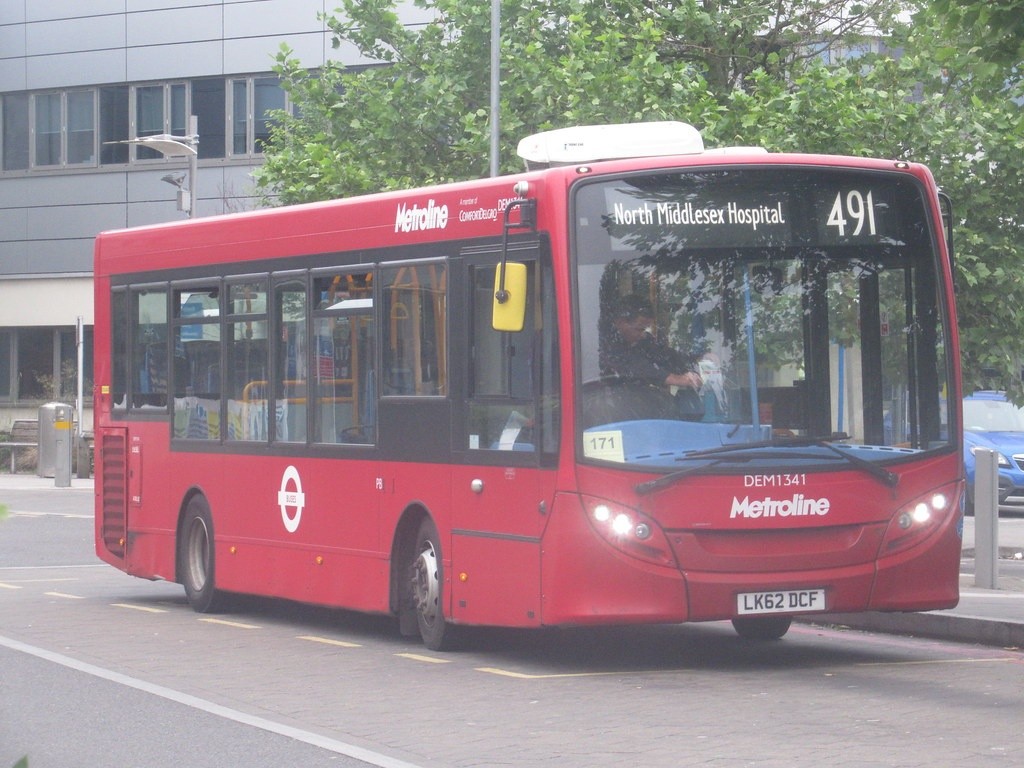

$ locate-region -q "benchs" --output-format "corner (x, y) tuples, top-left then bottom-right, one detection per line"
(0, 419), (78, 474)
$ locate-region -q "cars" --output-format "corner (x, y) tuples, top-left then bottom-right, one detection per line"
(881, 388), (1024, 515)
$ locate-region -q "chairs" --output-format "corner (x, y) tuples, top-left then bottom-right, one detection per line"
(141, 339), (416, 444)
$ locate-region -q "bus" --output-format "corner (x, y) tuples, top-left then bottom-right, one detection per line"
(94, 123), (965, 651)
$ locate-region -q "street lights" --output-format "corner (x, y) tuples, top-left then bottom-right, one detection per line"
(99, 134), (198, 219)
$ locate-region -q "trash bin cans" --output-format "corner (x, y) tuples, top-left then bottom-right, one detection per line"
(36, 402), (74, 478)
